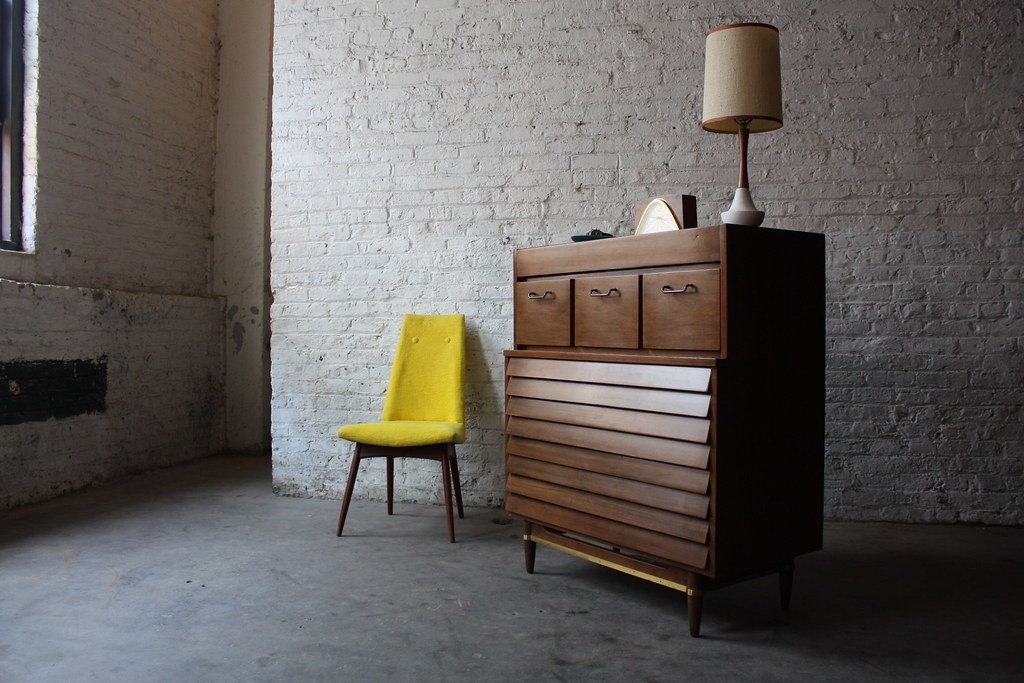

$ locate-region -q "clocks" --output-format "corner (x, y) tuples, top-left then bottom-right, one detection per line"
(504, 226), (829, 636)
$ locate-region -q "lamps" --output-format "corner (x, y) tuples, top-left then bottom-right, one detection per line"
(703, 23), (785, 226)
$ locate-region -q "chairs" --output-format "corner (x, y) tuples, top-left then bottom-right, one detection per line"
(334, 313), (469, 544)
